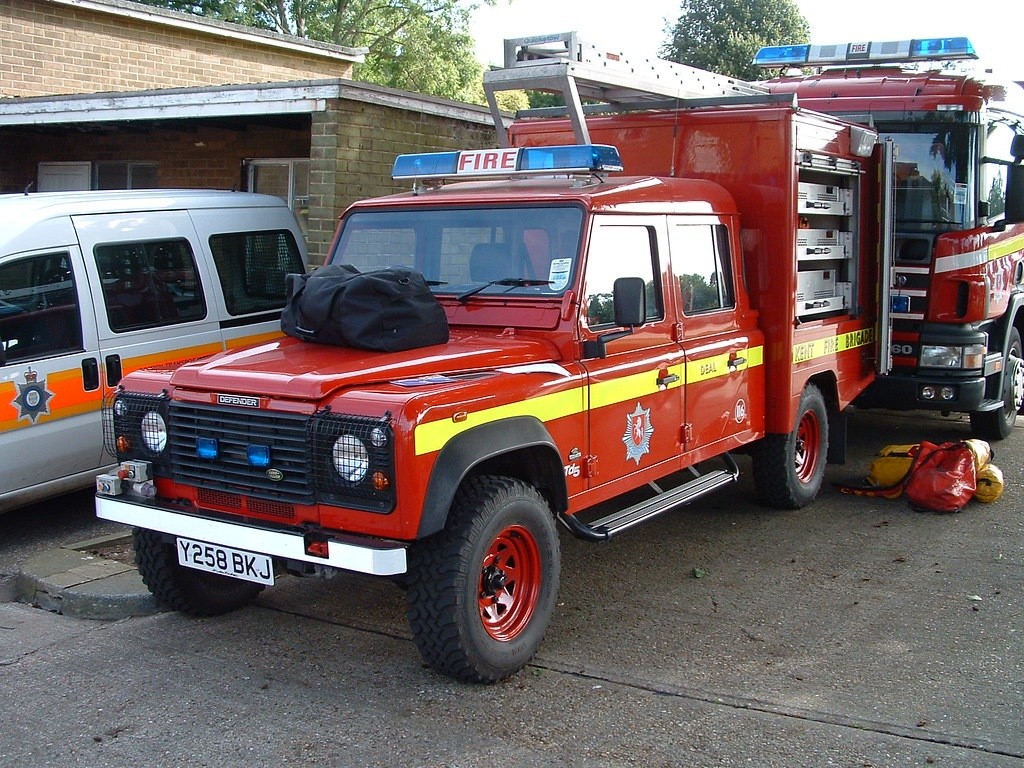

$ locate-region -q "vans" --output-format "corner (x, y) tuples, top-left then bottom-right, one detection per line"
(1, 189), (311, 519)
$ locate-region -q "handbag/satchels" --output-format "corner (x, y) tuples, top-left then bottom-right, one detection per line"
(280, 265), (451, 353)
(904, 440), (976, 511)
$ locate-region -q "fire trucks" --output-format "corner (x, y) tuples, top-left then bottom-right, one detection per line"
(749, 37), (1023, 443)
(94, 32), (894, 684)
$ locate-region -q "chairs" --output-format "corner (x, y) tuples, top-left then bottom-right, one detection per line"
(468, 244), (525, 285)
